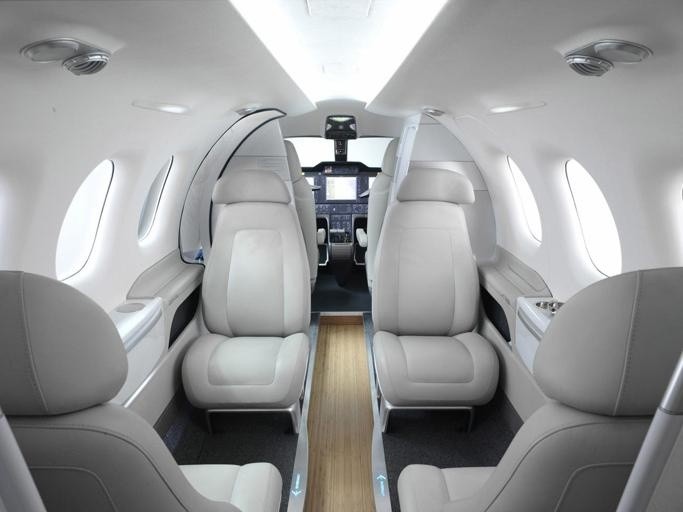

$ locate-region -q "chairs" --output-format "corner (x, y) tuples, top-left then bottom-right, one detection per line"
(283, 140), (326, 294)
(0, 270), (282, 512)
(182, 168), (311, 434)
(397, 267), (683, 512)
(356, 138), (399, 293)
(372, 168), (499, 436)
